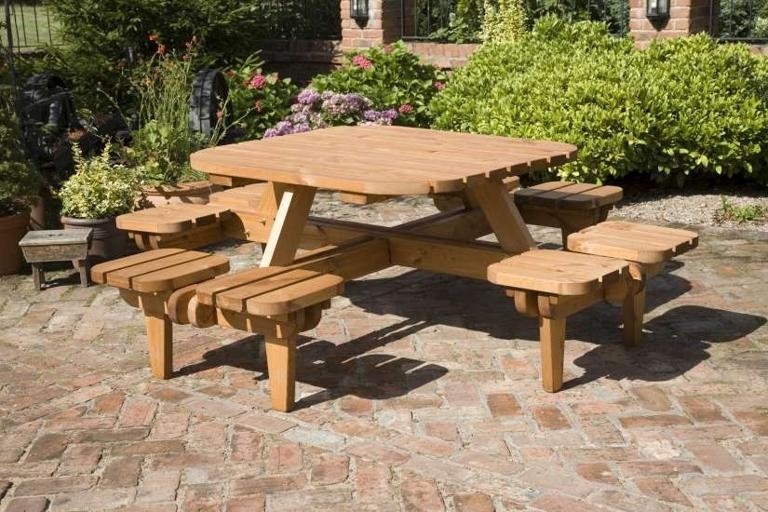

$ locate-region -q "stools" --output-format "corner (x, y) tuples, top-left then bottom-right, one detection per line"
(195, 266), (344, 411)
(19, 229), (93, 291)
(567, 220), (698, 343)
(210, 182), (267, 254)
(486, 250), (630, 392)
(514, 181), (623, 250)
(91, 248), (229, 379)
(116, 206), (231, 251)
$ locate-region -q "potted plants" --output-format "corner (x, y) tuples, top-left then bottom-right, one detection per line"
(132, 71), (213, 210)
(60, 137), (143, 258)
(0, 106), (39, 275)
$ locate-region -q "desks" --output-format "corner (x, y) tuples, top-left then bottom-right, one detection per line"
(190, 126), (578, 269)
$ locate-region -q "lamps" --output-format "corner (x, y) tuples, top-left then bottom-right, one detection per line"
(350, 1), (369, 21)
(646, 0), (670, 19)
